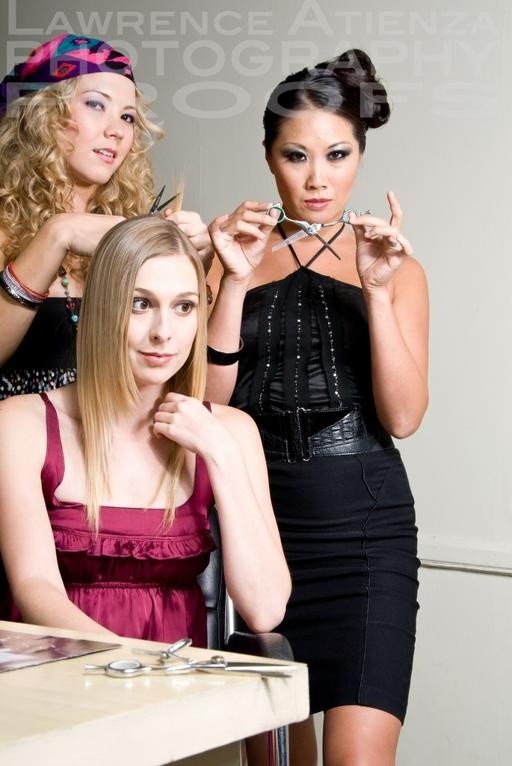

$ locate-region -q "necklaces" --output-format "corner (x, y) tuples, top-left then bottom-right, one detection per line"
(59, 265), (79, 330)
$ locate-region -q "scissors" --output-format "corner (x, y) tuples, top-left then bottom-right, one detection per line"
(149, 185), (182, 213)
(84, 638), (293, 678)
(266, 207), (359, 260)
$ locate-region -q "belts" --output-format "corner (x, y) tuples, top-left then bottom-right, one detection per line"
(256, 407), (395, 464)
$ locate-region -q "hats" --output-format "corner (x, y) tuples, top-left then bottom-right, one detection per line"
(0, 33), (136, 120)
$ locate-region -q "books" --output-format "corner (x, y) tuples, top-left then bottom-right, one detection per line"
(0, 630), (124, 677)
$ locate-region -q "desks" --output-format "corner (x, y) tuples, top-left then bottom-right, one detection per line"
(0, 617), (311, 766)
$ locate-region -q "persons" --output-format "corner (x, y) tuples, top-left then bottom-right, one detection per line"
(194, 44), (432, 766)
(0, 32), (215, 622)
(0, 213), (294, 643)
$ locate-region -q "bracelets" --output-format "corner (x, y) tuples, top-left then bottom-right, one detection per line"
(203, 337), (246, 367)
(0, 261), (50, 311)
